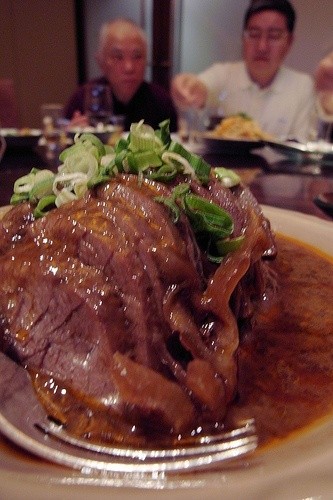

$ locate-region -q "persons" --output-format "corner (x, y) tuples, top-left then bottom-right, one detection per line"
(167, 0), (332, 146)
(59, 19), (181, 134)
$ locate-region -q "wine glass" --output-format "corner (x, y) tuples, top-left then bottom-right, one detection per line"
(84, 85), (112, 130)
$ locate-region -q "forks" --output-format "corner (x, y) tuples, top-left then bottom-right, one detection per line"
(0, 350), (258, 477)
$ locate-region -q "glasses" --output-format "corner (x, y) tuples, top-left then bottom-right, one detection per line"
(242, 29), (288, 43)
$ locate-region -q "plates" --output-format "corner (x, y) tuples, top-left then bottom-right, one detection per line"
(0, 204), (333, 500)
(66, 129), (114, 145)
(0, 129), (43, 147)
(202, 137), (265, 152)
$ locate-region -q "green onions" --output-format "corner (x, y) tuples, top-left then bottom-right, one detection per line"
(9, 120), (245, 262)
